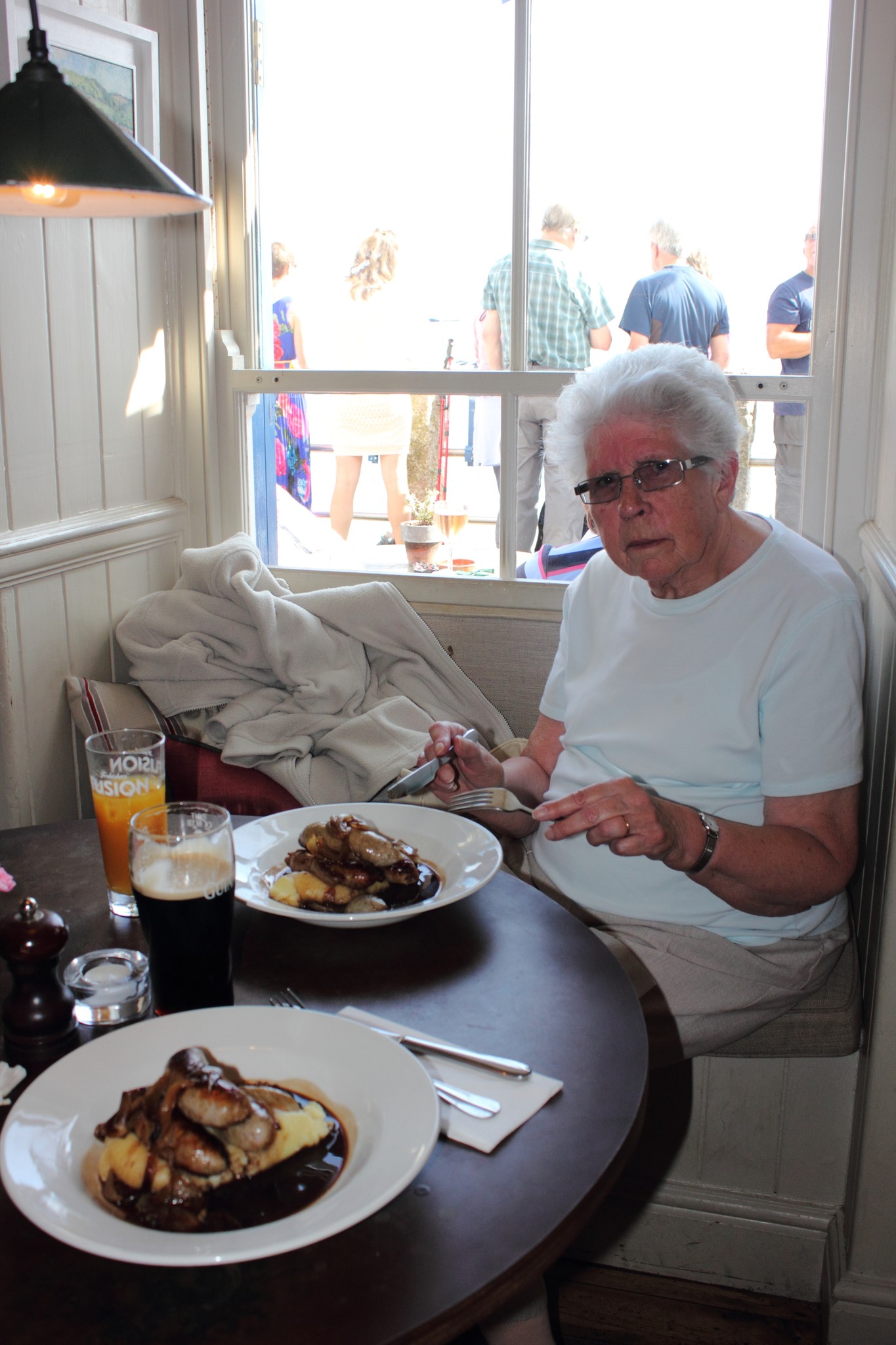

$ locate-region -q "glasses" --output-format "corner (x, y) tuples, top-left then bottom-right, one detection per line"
(574, 455), (714, 504)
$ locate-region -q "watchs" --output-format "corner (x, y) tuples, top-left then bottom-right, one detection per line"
(682, 811), (720, 876)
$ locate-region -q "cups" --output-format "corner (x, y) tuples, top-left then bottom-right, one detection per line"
(129, 801), (237, 1017)
(82, 726), (169, 917)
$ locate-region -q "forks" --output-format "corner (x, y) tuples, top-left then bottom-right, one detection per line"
(269, 988), (499, 1120)
(447, 786), (534, 815)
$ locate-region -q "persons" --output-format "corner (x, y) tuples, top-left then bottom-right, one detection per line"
(271, 241), (313, 513)
(687, 248), (750, 513)
(766, 221), (823, 533)
(475, 203), (614, 556)
(322, 232), (412, 546)
(617, 218), (733, 372)
(413, 342), (866, 1345)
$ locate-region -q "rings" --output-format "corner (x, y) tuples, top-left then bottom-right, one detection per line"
(621, 815), (630, 837)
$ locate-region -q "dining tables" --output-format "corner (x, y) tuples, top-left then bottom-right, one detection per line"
(0, 808), (684, 1345)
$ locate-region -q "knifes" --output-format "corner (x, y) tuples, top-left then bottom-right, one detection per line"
(388, 729), (482, 800)
(372, 1024), (530, 1080)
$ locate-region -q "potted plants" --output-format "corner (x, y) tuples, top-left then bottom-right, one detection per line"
(398, 490), (447, 569)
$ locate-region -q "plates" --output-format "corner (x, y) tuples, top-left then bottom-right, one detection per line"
(0, 1005), (447, 1267)
(229, 803), (504, 931)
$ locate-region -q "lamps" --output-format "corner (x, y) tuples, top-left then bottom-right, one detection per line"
(0, 0), (213, 222)
